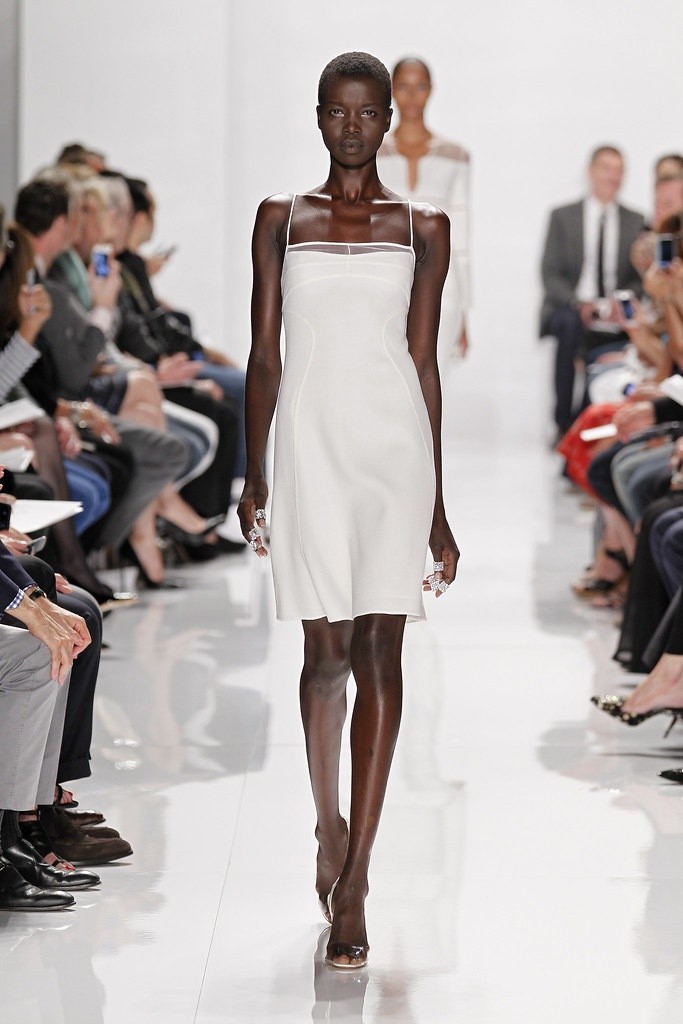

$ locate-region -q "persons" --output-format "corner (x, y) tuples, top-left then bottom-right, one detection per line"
(1, 145), (244, 911)
(540, 147), (682, 785)
(237, 51), (461, 969)
(375, 58), (468, 387)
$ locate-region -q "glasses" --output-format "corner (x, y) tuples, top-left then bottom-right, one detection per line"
(656, 170), (683, 183)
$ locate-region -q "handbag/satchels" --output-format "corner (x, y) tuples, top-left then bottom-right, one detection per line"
(115, 305), (203, 369)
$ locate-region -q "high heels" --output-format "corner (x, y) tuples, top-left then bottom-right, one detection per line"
(112, 537), (188, 591)
(157, 509), (225, 536)
(592, 696), (683, 739)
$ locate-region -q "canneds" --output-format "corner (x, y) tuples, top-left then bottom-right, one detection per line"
(90, 243), (114, 278)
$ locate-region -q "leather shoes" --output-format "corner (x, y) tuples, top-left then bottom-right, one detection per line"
(0, 839), (101, 910)
(40, 808), (132, 866)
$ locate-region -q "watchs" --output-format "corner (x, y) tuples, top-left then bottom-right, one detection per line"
(29, 588), (47, 600)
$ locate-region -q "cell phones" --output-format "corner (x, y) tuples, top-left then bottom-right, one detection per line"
(613, 289), (636, 326)
(27, 536), (46, 556)
(92, 245), (110, 278)
(624, 383), (635, 396)
(27, 268), (39, 315)
(658, 240), (674, 270)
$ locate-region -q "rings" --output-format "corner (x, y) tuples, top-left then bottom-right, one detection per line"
(431, 582), (439, 591)
(437, 581), (450, 594)
(249, 529), (261, 540)
(255, 509), (266, 520)
(249, 541), (257, 551)
(433, 561), (444, 571)
(429, 575), (435, 584)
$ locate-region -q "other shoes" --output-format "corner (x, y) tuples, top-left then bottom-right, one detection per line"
(162, 537), (246, 568)
(622, 658), (650, 673)
(657, 768), (683, 783)
(568, 480), (596, 512)
(572, 564), (628, 609)
(98, 592), (139, 612)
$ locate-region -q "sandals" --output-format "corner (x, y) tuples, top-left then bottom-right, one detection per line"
(327, 875), (369, 968)
(37, 785), (78, 809)
(319, 892), (334, 925)
(19, 821), (75, 872)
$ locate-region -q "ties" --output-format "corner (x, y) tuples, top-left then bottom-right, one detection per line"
(599, 214), (604, 297)
